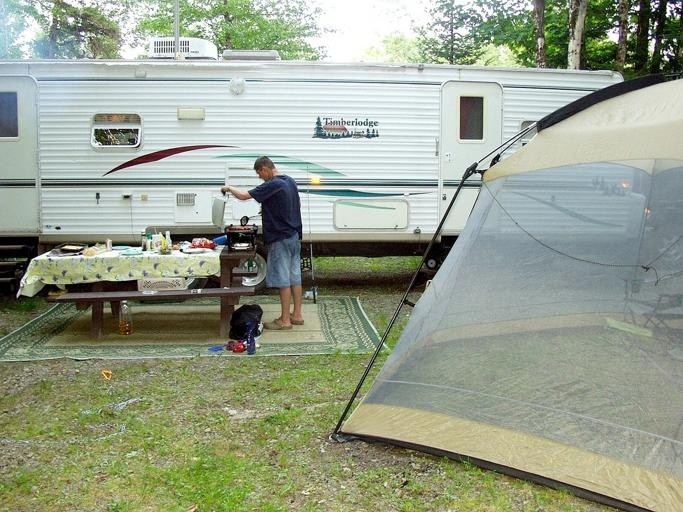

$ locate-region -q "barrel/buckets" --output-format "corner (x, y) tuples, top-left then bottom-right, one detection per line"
(264, 318), (293, 330)
(289, 312), (304, 325)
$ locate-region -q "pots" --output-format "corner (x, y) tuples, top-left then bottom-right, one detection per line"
(223, 225), (260, 243)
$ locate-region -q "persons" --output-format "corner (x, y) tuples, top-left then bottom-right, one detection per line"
(220, 154), (304, 330)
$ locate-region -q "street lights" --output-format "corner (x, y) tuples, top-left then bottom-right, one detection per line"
(229, 304), (263, 340)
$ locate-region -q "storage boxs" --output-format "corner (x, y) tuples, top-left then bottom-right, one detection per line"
(47, 265), (258, 338)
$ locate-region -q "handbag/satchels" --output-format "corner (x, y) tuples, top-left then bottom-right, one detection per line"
(227, 186), (231, 193)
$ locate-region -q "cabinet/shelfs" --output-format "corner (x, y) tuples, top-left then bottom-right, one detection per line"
(246, 324), (255, 355)
(118, 299), (134, 335)
(95, 229), (216, 254)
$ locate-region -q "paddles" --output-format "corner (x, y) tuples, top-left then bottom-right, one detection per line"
(0, 295), (390, 362)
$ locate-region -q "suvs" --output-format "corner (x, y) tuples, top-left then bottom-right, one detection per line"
(118, 301), (134, 336)
